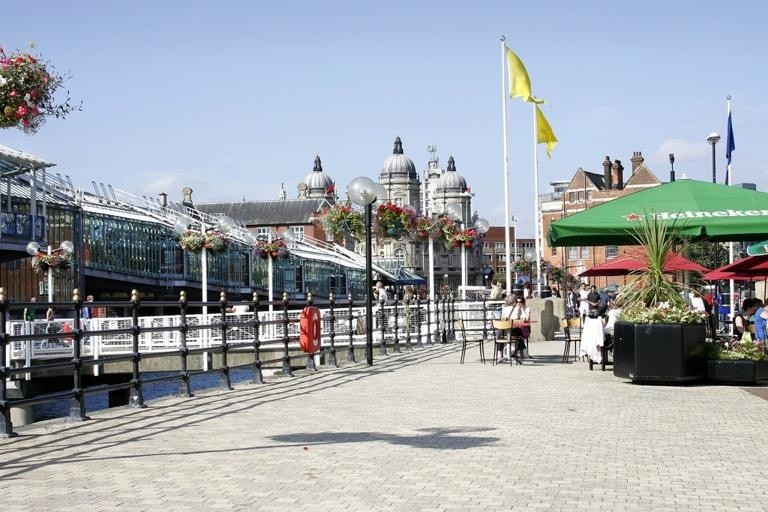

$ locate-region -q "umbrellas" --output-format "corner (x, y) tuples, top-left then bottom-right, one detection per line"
(581, 239), (768, 304)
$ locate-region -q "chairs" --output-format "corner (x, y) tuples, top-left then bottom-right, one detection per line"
(561, 313), (614, 371)
(458, 319), (529, 367)
(710, 314), (738, 344)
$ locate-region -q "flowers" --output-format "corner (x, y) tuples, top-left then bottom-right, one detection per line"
(176, 228), (228, 257)
(251, 238), (290, 258)
(705, 336), (768, 362)
(0, 39), (84, 135)
(36, 251), (72, 273)
(613, 302), (710, 325)
(316, 200), (485, 253)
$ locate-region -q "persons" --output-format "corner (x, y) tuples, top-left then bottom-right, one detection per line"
(498, 294), (524, 365)
(225, 304), (239, 323)
(552, 283), (622, 349)
(80, 293), (94, 345)
(733, 299), (768, 342)
(373, 282), (424, 302)
(233, 299), (250, 312)
(515, 295), (530, 360)
(23, 296), (38, 348)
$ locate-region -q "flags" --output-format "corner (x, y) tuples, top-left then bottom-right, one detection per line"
(725, 109), (733, 166)
(508, 49), (547, 104)
(535, 104), (557, 158)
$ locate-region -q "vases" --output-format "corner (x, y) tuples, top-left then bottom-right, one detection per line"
(706, 359), (768, 386)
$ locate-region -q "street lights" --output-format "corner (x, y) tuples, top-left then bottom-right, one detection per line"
(707, 133), (721, 298)
(245, 228), (298, 340)
(474, 216), (489, 260)
(175, 214), (233, 373)
(524, 248), (537, 296)
(26, 239), (74, 303)
(349, 177), (387, 366)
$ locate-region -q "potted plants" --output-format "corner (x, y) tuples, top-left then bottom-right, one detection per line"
(608, 205), (713, 385)
